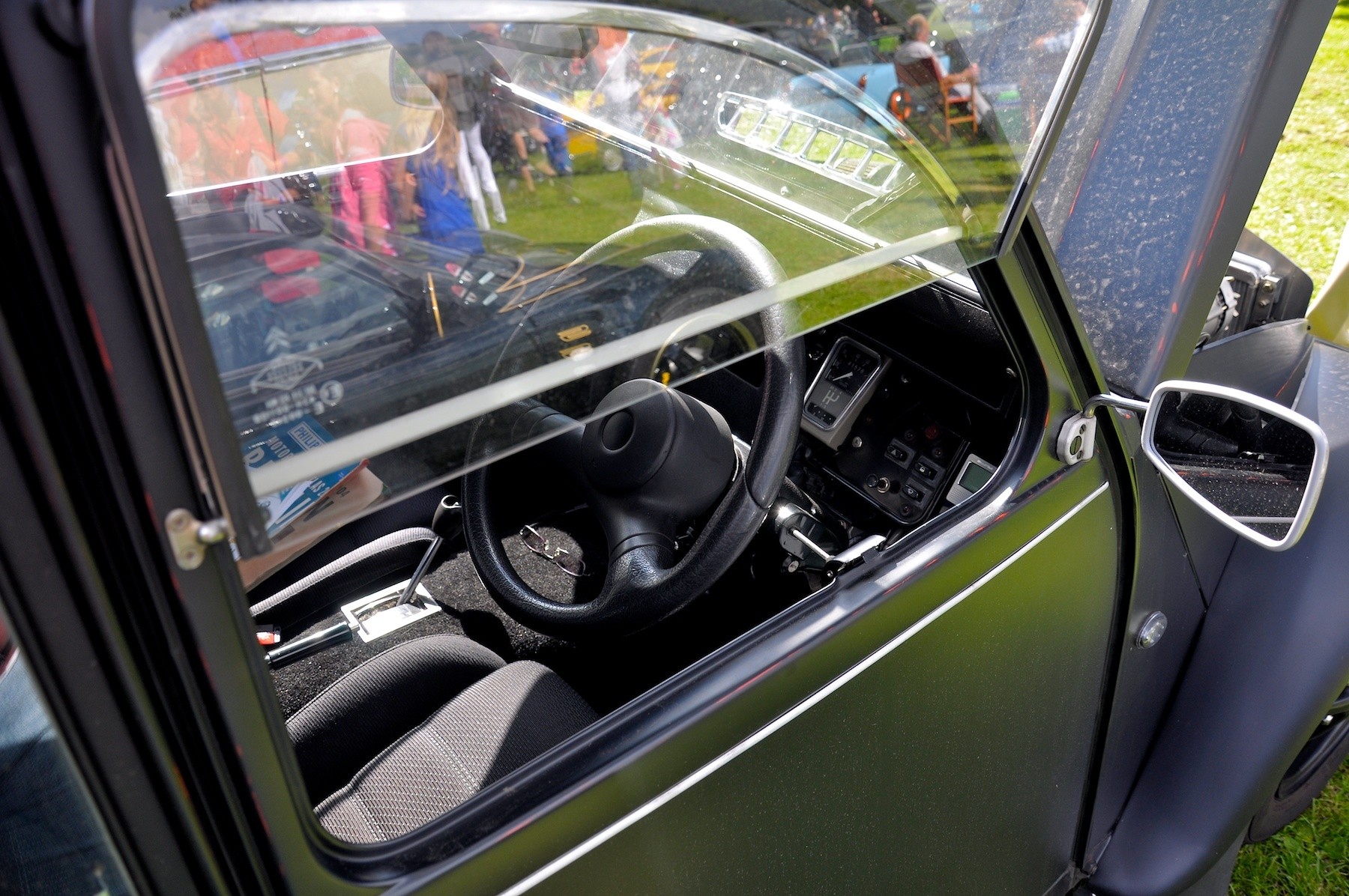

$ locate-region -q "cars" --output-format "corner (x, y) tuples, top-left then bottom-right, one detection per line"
(0, 0), (1349, 896)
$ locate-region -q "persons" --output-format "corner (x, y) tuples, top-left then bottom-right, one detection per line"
(783, 0), (885, 59)
(895, 15), (993, 125)
(192, 27), (688, 257)
(1029, 0), (1094, 58)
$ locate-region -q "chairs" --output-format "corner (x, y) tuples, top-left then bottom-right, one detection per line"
(891, 55), (978, 146)
(285, 631), (592, 850)
